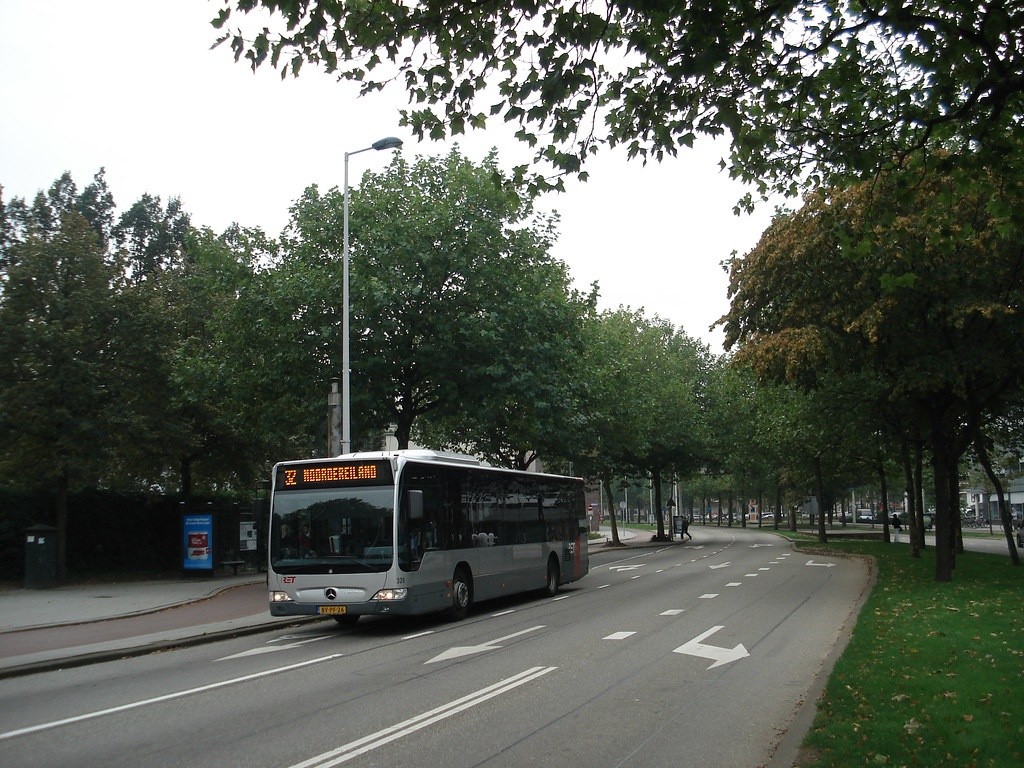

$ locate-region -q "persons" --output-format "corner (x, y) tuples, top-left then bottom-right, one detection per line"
(280, 524), (290, 549)
(1011, 509), (1019, 531)
(892, 514), (900, 542)
(681, 516), (692, 540)
(298, 522), (311, 548)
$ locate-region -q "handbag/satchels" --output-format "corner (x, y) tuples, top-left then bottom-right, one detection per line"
(898, 526), (903, 532)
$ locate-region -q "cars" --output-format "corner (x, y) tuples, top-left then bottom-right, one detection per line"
(1016, 526), (1024, 548)
(704, 507), (902, 521)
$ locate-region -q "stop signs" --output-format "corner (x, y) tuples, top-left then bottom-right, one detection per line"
(588, 505), (593, 512)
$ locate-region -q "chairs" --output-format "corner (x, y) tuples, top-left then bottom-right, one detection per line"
(441, 519), (545, 551)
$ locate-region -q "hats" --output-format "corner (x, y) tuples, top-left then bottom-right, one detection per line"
(892, 514), (897, 516)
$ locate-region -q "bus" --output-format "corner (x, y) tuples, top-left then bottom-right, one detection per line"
(267, 450), (590, 627)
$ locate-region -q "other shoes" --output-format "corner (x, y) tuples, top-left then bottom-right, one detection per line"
(689, 536), (692, 540)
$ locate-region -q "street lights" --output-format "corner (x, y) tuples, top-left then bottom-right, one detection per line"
(341, 136), (404, 450)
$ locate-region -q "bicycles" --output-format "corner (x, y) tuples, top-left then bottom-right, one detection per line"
(1000, 521), (1015, 532)
(964, 515), (990, 529)
